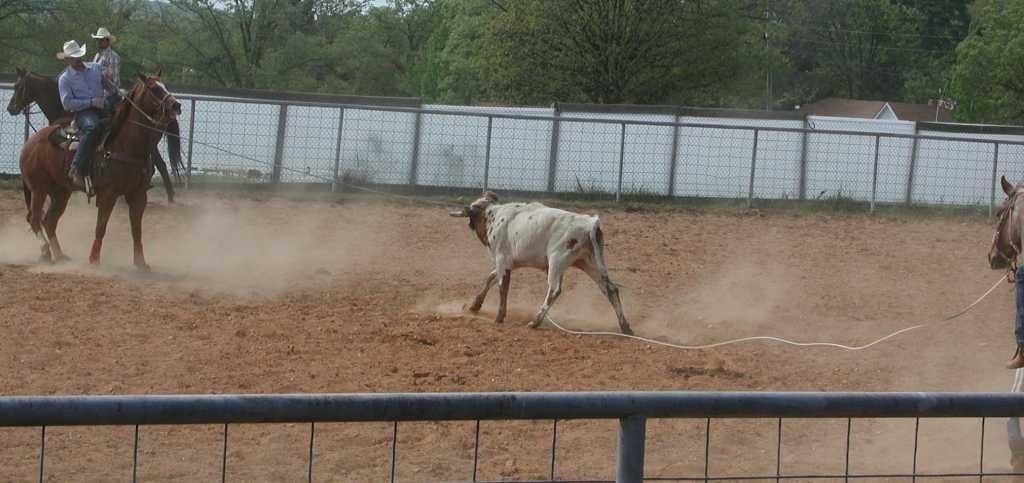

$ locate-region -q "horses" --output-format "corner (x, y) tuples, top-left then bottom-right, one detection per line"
(987, 175), (1024, 270)
(19, 66), (181, 270)
(7, 64), (188, 205)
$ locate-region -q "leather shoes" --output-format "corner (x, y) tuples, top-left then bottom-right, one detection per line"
(67, 171), (80, 189)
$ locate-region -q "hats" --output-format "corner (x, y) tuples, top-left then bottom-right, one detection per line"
(91, 28), (116, 43)
(56, 40), (87, 60)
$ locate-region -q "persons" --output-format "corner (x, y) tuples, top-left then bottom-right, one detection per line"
(90, 28), (121, 104)
(1007, 267), (1023, 366)
(1005, 416), (1024, 483)
(56, 39), (112, 189)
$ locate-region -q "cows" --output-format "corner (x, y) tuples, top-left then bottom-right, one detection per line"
(449, 190), (634, 335)
